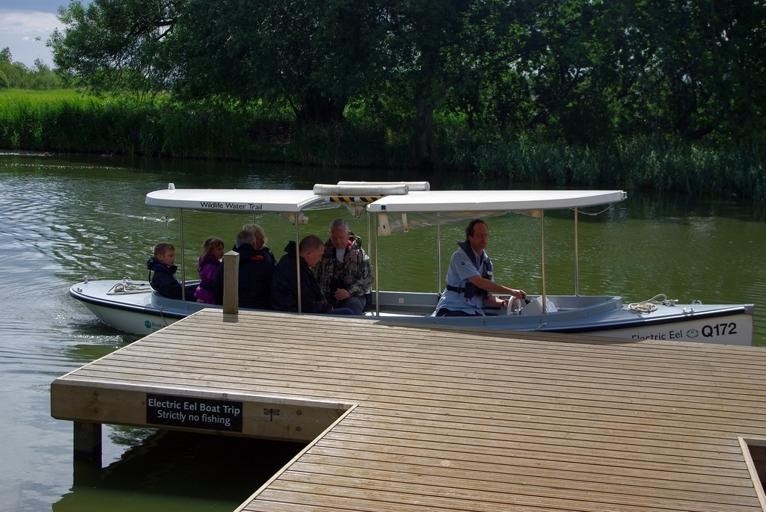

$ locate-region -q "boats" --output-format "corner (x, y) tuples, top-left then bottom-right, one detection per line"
(67, 180), (755, 344)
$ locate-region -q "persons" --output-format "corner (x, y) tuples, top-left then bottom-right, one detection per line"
(193, 236), (224, 304)
(146, 243), (196, 302)
(242, 223), (278, 266)
(435, 218), (528, 317)
(264, 234), (355, 313)
(310, 218), (374, 314)
(233, 230), (275, 307)
